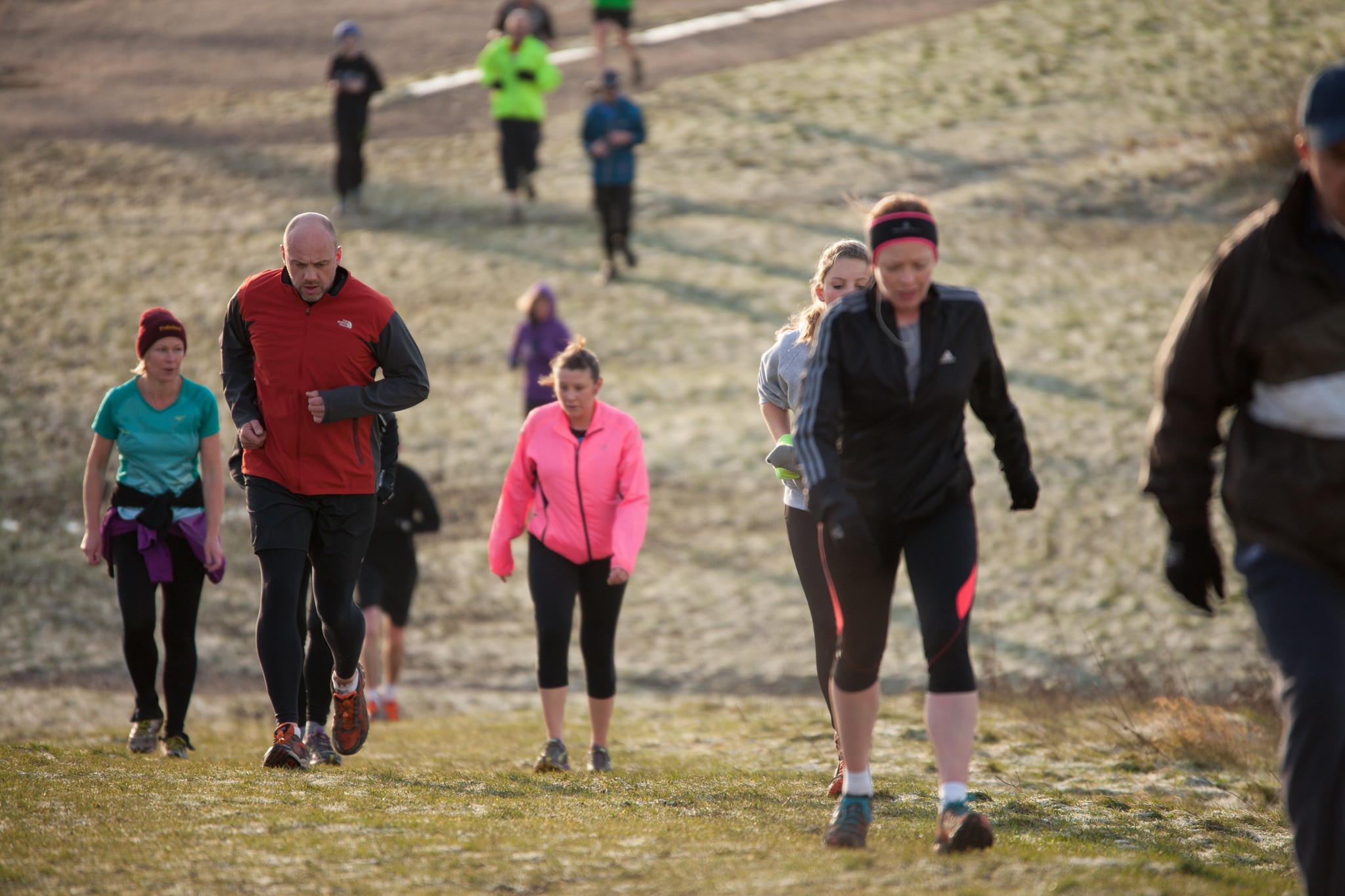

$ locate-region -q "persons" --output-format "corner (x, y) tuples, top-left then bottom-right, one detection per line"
(351, 460), (442, 721)
(228, 431), (342, 767)
(80, 308), (225, 761)
(477, 7), (560, 214)
(488, 0), (555, 49)
(589, 0), (646, 93)
(1142, 69), (1345, 894)
(220, 212), (429, 771)
(579, 72), (645, 280)
(797, 195), (1042, 852)
(507, 285), (572, 416)
(761, 240), (874, 801)
(326, 20), (388, 221)
(486, 335), (650, 771)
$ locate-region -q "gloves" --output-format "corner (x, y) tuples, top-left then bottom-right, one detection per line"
(1165, 535), (1227, 613)
(773, 433), (799, 480)
(826, 507), (880, 568)
(1003, 465), (1040, 511)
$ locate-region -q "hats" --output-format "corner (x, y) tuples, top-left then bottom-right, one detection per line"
(136, 307), (186, 358)
(1299, 65), (1344, 148)
(765, 444), (805, 491)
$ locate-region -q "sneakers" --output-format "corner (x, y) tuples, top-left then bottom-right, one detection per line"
(159, 734), (189, 759)
(302, 728), (342, 767)
(823, 794), (873, 853)
(128, 716), (164, 752)
(260, 723), (314, 771)
(588, 743), (613, 774)
(330, 661), (370, 756)
(825, 757), (844, 797)
(934, 801), (994, 856)
(533, 739), (572, 773)
